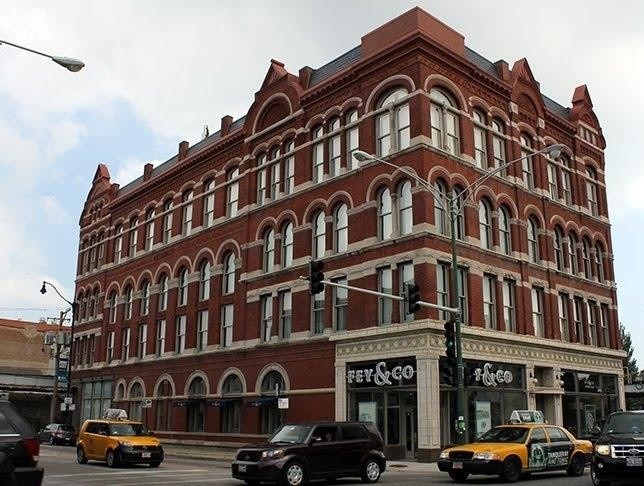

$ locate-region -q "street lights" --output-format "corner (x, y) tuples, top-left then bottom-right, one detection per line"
(351, 143), (570, 448)
(40, 277), (82, 425)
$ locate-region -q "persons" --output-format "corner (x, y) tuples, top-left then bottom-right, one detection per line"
(325, 431), (333, 442)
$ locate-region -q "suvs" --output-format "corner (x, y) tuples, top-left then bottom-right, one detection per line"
(0, 389), (46, 485)
(37, 422), (78, 447)
(75, 407), (166, 469)
(229, 419), (388, 485)
(588, 409), (643, 486)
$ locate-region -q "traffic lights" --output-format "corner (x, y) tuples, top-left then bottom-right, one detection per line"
(403, 280), (422, 317)
(442, 318), (458, 368)
(308, 260), (326, 297)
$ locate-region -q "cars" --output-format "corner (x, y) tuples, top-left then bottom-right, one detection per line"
(438, 409), (596, 484)
